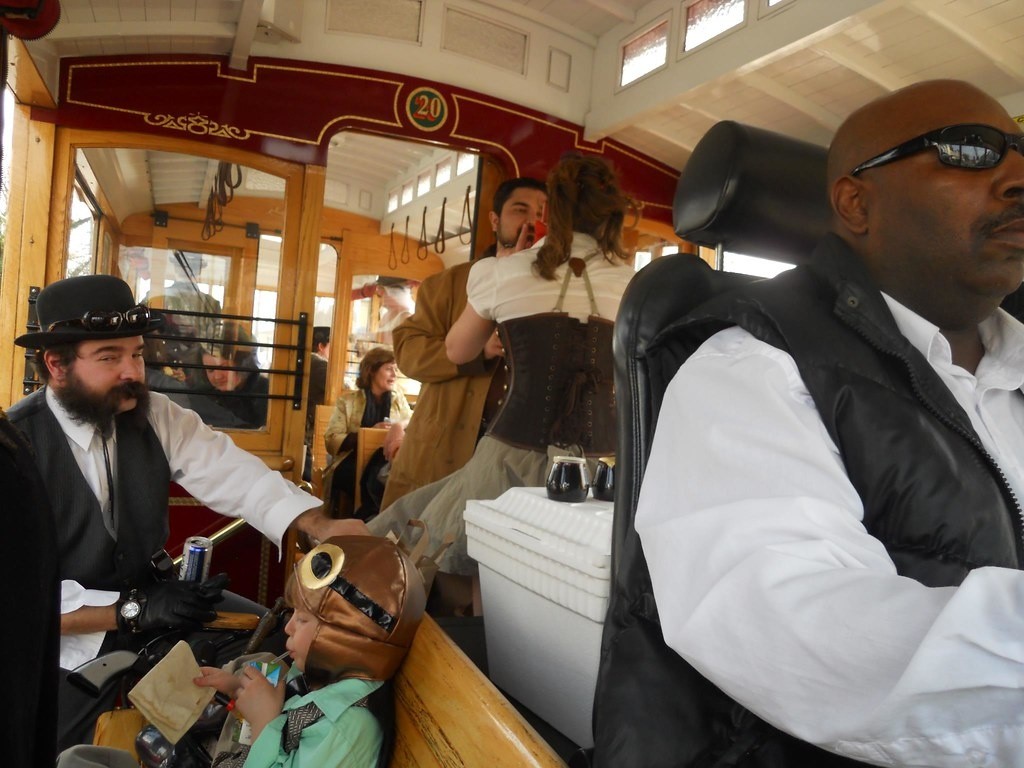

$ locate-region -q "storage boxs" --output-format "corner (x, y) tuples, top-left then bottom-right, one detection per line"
(462, 485), (615, 753)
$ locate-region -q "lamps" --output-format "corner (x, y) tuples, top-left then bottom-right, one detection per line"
(246, 222), (259, 239)
(154, 211), (169, 227)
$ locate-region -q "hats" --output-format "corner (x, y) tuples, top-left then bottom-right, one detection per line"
(15, 275), (166, 349)
(170, 251), (207, 269)
(199, 323), (252, 358)
(282, 534), (428, 680)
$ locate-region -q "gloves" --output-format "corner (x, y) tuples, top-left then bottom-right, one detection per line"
(116, 572), (230, 634)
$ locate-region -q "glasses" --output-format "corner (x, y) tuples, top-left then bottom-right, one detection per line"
(47, 304), (151, 332)
(850, 123), (1024, 177)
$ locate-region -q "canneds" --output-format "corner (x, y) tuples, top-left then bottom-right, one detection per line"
(178, 535), (213, 584)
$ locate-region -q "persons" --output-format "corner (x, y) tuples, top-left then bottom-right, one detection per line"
(193, 535), (427, 768)
(366, 152), (635, 579)
(0, 250), (415, 768)
(589, 81), (1024, 768)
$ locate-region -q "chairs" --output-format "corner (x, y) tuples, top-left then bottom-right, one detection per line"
(590, 116), (837, 768)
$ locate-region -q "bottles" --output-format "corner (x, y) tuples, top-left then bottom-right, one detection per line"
(592, 456), (616, 502)
(546, 454), (592, 501)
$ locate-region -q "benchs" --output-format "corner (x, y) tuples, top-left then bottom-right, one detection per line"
(60, 525), (570, 768)
(311, 403), (408, 519)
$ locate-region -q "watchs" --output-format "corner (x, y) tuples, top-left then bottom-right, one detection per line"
(116, 588), (148, 634)
